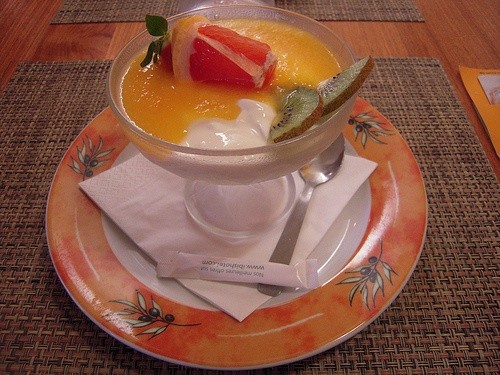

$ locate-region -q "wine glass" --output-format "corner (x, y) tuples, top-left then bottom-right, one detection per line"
(105, 4), (363, 237)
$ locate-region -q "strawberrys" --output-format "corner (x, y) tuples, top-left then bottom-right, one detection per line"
(143, 16), (279, 91)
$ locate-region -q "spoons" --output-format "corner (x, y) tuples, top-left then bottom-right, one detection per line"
(257, 132), (346, 296)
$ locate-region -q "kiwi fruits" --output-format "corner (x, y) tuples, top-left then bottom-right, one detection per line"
(268, 56), (374, 143)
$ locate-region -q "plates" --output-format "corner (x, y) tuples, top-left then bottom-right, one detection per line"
(46, 96), (429, 374)
